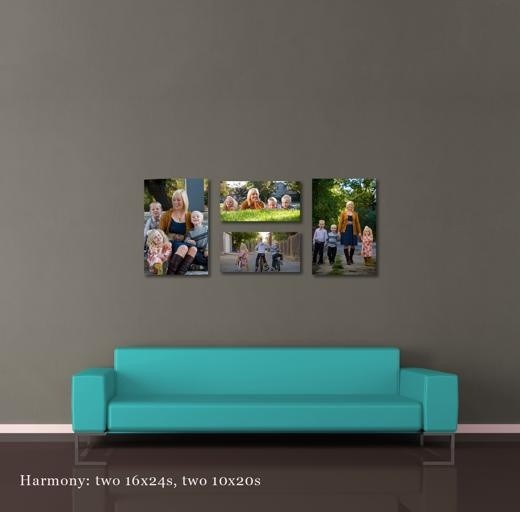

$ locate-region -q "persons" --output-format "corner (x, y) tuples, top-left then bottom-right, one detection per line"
(142, 179), (210, 276)
(268, 241), (284, 271)
(253, 237), (271, 272)
(337, 201), (362, 265)
(234, 243), (250, 271)
(358, 226), (374, 265)
(312, 220), (329, 265)
(221, 185), (296, 213)
(326, 224), (342, 265)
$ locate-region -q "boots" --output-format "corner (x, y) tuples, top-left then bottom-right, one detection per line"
(344, 248), (355, 266)
(176, 255), (194, 275)
(167, 254), (184, 275)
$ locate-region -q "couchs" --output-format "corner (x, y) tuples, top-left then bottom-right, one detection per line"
(71, 347), (459, 468)
(74, 447), (457, 511)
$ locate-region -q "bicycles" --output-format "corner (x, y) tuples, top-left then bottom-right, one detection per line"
(271, 250), (282, 272)
(233, 253), (247, 272)
(256, 251), (267, 272)
